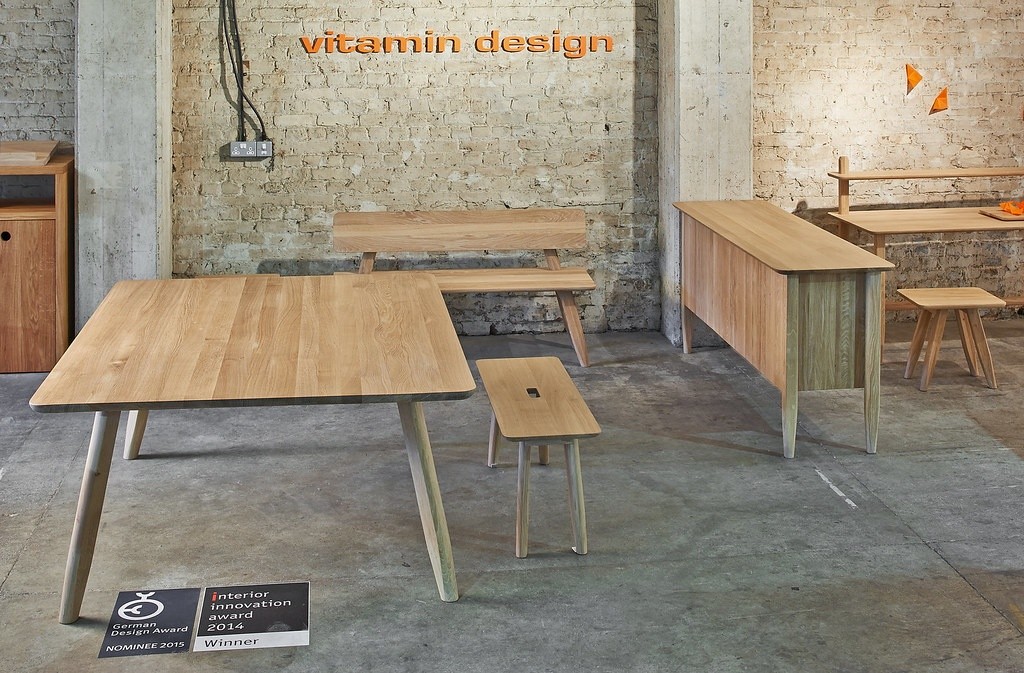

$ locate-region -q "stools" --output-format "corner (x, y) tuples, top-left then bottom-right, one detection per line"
(898, 287), (1006, 391)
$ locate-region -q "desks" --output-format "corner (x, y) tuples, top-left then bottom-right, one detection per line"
(331, 209), (592, 367)
(30, 276), (476, 624)
(674, 200), (894, 457)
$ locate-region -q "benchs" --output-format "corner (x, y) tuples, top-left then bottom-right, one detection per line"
(335, 267), (598, 366)
(476, 355), (602, 559)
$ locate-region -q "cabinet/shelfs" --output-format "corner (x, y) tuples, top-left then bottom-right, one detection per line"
(827, 154), (1024, 308)
(1, 154), (75, 373)
